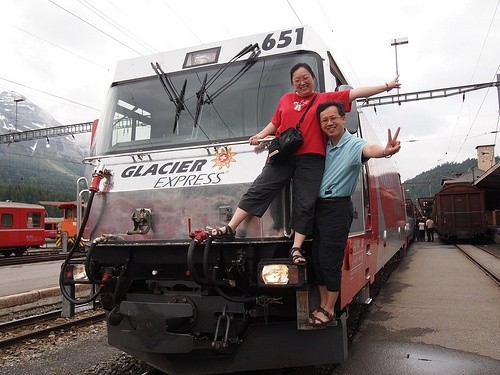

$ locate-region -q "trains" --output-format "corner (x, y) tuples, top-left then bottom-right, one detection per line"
(429, 181), (487, 243)
(0, 199), (86, 258)
(57, 20), (419, 375)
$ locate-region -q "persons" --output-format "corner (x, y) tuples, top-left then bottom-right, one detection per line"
(425, 216), (435, 242)
(303, 101), (401, 326)
(416, 220), (425, 241)
(210, 62), (402, 266)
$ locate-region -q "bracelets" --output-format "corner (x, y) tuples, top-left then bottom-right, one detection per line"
(383, 150), (392, 159)
(385, 83), (390, 92)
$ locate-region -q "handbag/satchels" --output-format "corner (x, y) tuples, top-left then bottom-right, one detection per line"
(267, 126), (304, 159)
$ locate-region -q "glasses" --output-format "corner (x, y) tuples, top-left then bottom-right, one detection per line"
(319, 115), (342, 123)
(292, 74), (311, 84)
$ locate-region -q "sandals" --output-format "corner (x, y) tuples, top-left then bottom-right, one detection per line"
(209, 224), (237, 241)
(289, 247), (308, 266)
(307, 306), (337, 328)
